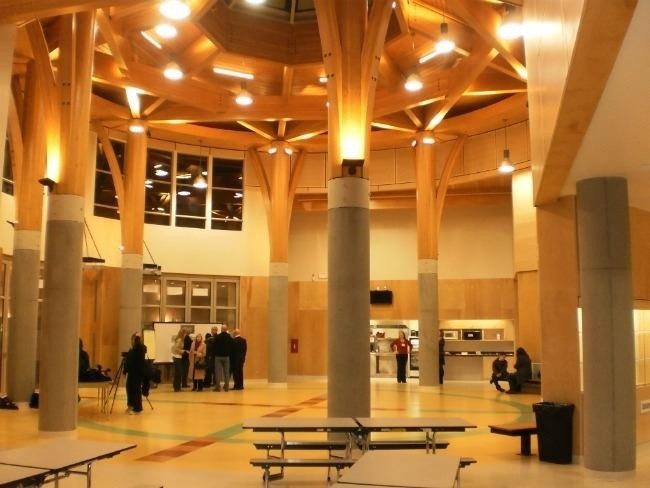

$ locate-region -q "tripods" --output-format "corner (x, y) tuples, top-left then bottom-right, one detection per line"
(102, 360), (157, 413)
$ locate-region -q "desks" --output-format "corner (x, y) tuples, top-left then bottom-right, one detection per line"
(242, 413), (361, 487)
(333, 449), (466, 487)
(73, 379), (111, 419)
(355, 413), (479, 476)
(329, 481), (398, 487)
(0, 457), (52, 487)
(0, 438), (139, 487)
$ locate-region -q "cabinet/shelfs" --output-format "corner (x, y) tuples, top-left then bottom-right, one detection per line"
(371, 323), (410, 360)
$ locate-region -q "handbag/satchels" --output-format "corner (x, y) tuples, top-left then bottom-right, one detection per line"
(195, 352), (207, 370)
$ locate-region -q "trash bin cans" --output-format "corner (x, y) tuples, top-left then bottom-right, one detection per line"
(532, 402), (575, 464)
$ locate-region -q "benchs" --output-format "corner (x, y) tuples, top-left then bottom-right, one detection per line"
(459, 457), (476, 464)
(247, 455), (354, 487)
(359, 438), (454, 451)
(253, 436), (356, 482)
(504, 372), (542, 385)
(487, 420), (541, 457)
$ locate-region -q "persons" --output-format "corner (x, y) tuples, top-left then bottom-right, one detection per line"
(78, 336), (90, 405)
(390, 330), (413, 382)
(172, 324), (247, 391)
(506, 346), (532, 393)
(438, 329), (445, 382)
(124, 334), (144, 415)
(490, 351), (509, 391)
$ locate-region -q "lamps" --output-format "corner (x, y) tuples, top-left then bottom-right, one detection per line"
(80, 219), (107, 268)
(36, 0), (521, 198)
(144, 240), (164, 273)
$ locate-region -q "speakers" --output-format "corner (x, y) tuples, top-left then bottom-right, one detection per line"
(370, 290), (392, 304)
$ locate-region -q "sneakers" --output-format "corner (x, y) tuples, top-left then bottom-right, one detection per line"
(489, 376), (493, 384)
(495, 385), (522, 394)
(173, 384), (245, 391)
(125, 405), (144, 415)
(396, 379), (408, 384)
